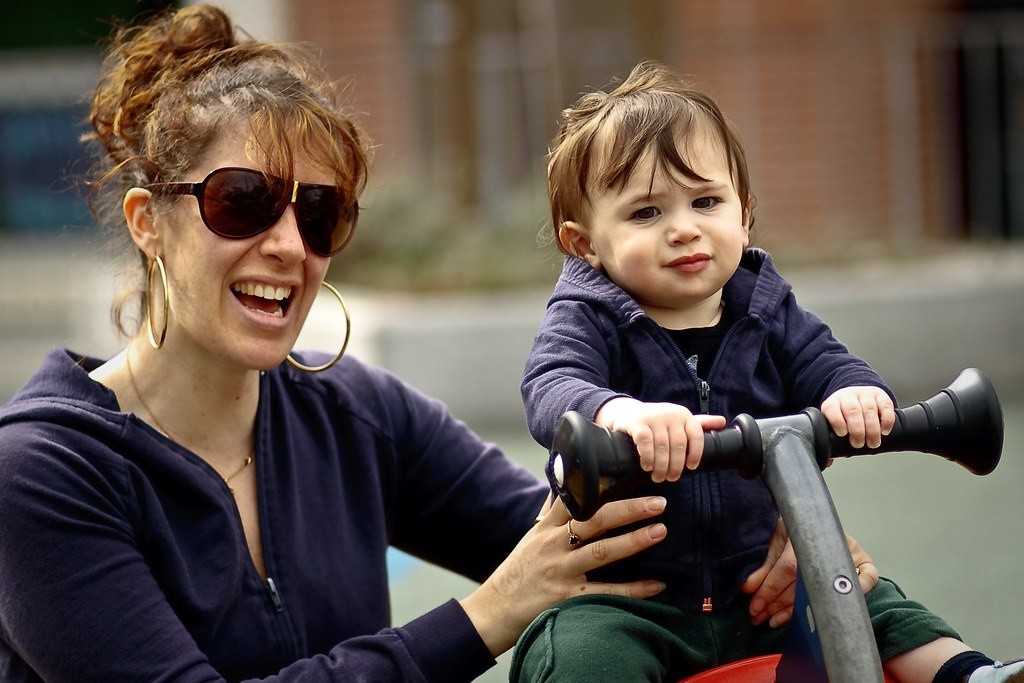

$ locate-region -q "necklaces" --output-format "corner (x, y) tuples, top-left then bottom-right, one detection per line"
(127, 347), (254, 497)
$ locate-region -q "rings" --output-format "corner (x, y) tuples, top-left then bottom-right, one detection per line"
(567, 520), (582, 546)
(855, 561), (873, 576)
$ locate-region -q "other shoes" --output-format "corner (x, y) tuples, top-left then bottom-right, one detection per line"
(967, 656), (1024, 683)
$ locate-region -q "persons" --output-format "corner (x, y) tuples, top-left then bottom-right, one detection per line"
(505, 63), (1024, 683)
(0, 5), (878, 683)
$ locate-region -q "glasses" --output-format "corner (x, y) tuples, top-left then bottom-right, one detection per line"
(142, 166), (361, 257)
